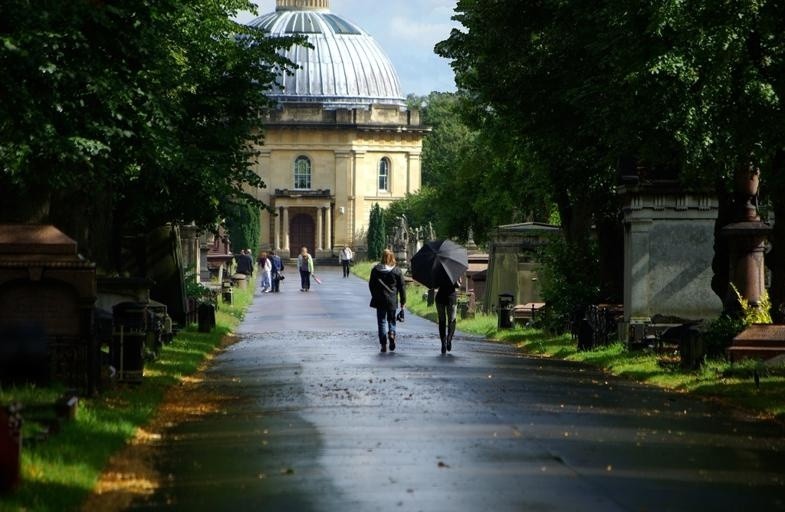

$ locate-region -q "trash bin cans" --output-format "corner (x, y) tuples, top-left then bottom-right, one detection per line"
(222, 280), (234, 305)
(498, 293), (515, 331)
(112, 301), (147, 384)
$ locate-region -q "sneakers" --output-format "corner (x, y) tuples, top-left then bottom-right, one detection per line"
(441, 342), (452, 354)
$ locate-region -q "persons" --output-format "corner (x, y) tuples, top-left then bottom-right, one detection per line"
(298, 247), (314, 291)
(237, 249), (284, 293)
(435, 276), (463, 353)
(339, 243), (353, 276)
(368, 249), (406, 352)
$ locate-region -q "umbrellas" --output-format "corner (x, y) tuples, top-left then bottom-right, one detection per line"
(410, 239), (469, 289)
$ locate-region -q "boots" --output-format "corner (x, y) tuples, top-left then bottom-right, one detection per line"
(388, 331), (396, 351)
(380, 335), (387, 353)
